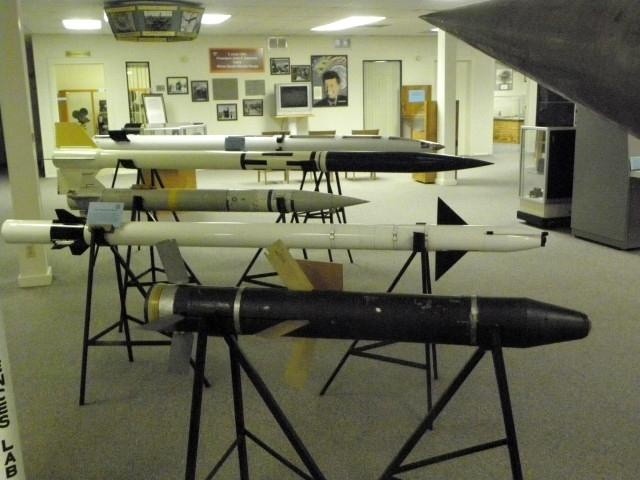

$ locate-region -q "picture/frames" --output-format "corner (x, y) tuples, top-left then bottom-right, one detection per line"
(243, 98), (263, 116)
(167, 76), (188, 95)
(269, 58), (290, 75)
(191, 81), (209, 102)
(310, 55), (349, 108)
(291, 65), (310, 82)
(217, 104), (238, 121)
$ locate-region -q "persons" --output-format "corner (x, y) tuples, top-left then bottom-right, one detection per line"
(313, 71), (347, 106)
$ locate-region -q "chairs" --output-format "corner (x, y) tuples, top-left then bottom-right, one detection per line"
(257, 129), (380, 184)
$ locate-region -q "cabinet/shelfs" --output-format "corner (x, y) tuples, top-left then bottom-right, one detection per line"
(516, 125), (575, 228)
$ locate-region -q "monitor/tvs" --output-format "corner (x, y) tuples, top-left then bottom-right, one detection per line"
(275, 83), (313, 116)
